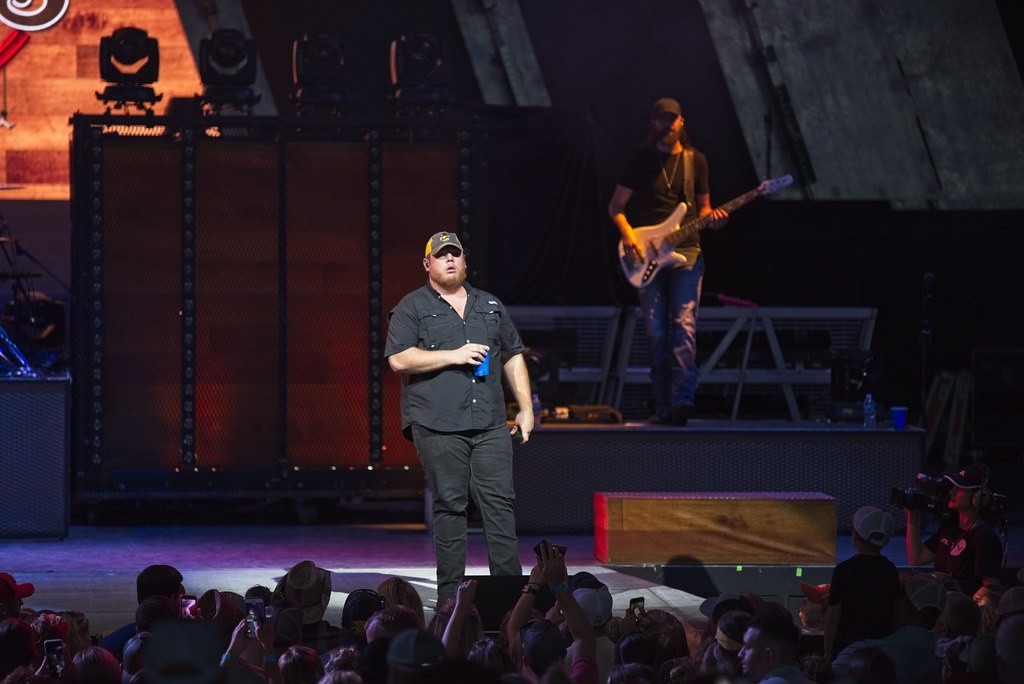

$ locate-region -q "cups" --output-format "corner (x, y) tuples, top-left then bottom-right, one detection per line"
(473, 347), (490, 375)
(890, 407), (907, 430)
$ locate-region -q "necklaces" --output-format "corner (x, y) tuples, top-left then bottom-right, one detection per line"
(654, 146), (681, 191)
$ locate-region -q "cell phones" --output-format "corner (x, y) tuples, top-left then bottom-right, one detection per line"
(630, 597), (644, 624)
(533, 541), (554, 561)
(44, 639), (66, 679)
(244, 597), (265, 639)
(180, 595), (197, 623)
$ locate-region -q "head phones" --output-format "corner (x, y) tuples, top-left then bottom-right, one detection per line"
(973, 470), (994, 513)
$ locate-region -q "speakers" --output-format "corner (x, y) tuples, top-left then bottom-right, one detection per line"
(461, 575), (556, 640)
(0, 367), (72, 541)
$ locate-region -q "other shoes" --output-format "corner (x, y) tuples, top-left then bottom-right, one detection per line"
(672, 400), (694, 428)
(649, 404), (672, 425)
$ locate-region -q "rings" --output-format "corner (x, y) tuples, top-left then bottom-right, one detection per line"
(718, 219), (721, 223)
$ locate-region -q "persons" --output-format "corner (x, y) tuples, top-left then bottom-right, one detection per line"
(608, 97), (730, 427)
(633, 605), (640, 618)
(0, 465), (1024, 684)
(51, 653), (60, 669)
(246, 608), (260, 633)
(384, 232), (535, 613)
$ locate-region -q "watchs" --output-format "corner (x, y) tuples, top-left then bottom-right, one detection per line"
(521, 584), (538, 595)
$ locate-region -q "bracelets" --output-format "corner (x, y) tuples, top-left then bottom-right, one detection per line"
(220, 652), (240, 665)
(262, 654), (277, 663)
(551, 581), (568, 596)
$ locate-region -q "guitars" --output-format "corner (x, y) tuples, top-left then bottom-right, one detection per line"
(618, 175), (796, 288)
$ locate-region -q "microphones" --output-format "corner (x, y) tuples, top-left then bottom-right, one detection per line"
(916, 474), (943, 487)
(924, 273), (933, 300)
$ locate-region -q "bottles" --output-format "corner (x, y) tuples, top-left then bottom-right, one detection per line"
(532, 394), (541, 430)
(863, 393), (875, 428)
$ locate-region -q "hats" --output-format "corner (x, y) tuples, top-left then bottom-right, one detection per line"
(0, 573), (34, 603)
(653, 97), (681, 116)
(568, 571), (613, 626)
(990, 586), (1024, 630)
(942, 463), (987, 489)
(850, 504), (895, 547)
(266, 599), (303, 642)
(137, 565), (186, 602)
(389, 630), (449, 671)
(959, 634), (997, 673)
(189, 589), (248, 643)
(341, 589), (384, 633)
(425, 232), (463, 258)
(121, 632), (157, 684)
(700, 592), (753, 626)
(934, 634), (975, 671)
(271, 560), (332, 625)
(899, 571), (947, 618)
